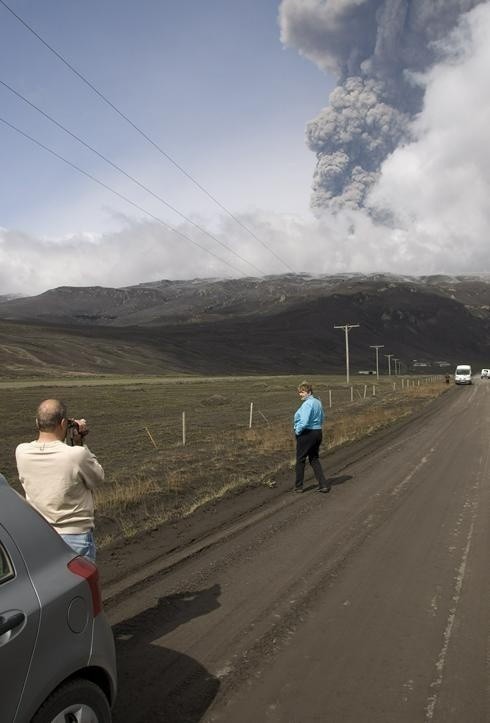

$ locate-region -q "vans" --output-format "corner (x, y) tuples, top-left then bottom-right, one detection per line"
(455, 364), (472, 385)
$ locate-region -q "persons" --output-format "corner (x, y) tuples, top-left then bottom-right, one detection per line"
(15, 399), (106, 563)
(289, 382), (331, 493)
(445, 373), (450, 383)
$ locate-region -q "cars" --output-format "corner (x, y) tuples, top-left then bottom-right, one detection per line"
(0, 476), (117, 720)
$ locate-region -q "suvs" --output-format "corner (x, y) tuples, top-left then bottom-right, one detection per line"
(480, 369), (490, 379)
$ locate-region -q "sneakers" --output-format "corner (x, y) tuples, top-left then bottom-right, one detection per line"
(316, 487), (329, 494)
(293, 488), (304, 493)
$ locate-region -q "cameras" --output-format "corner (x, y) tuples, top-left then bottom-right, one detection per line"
(66, 420), (88, 436)
